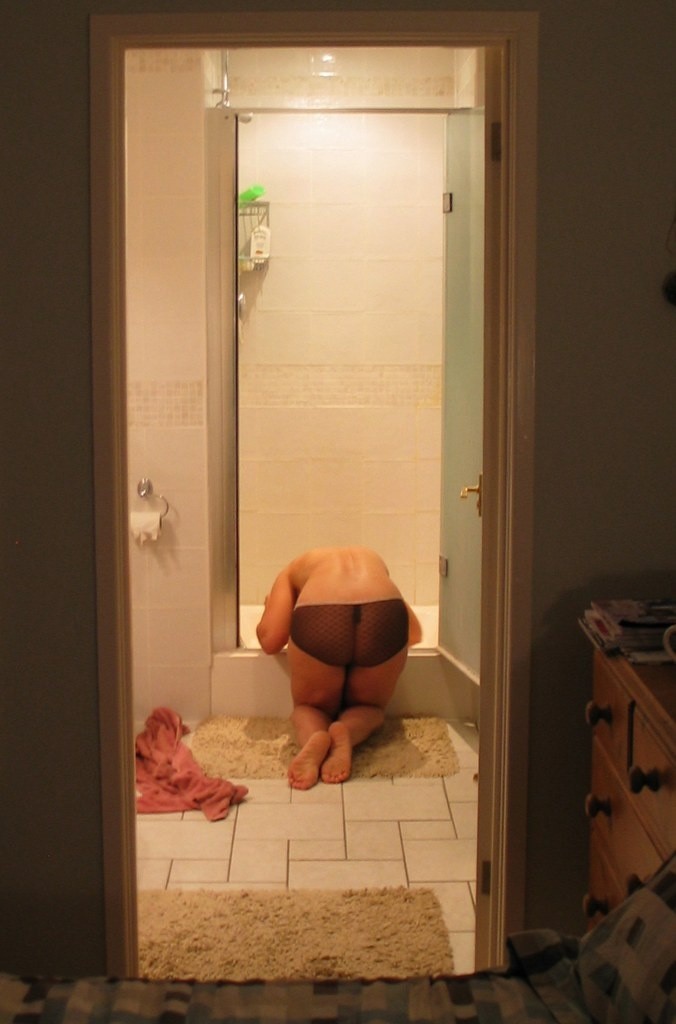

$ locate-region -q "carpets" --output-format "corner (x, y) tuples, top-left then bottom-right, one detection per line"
(136, 887), (454, 980)
(187, 715), (461, 781)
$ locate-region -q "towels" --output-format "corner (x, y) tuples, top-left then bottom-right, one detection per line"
(133, 703), (251, 826)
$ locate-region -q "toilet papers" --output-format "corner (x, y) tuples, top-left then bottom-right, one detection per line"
(127, 508), (166, 548)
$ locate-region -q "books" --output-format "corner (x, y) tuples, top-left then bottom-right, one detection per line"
(577, 596), (676, 654)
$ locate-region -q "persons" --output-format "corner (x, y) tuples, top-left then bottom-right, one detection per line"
(257, 546), (424, 791)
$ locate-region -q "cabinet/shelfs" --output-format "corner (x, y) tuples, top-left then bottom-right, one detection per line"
(580, 618), (676, 933)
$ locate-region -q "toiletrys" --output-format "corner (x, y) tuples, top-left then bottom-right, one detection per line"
(250, 224), (272, 271)
(237, 183), (267, 212)
